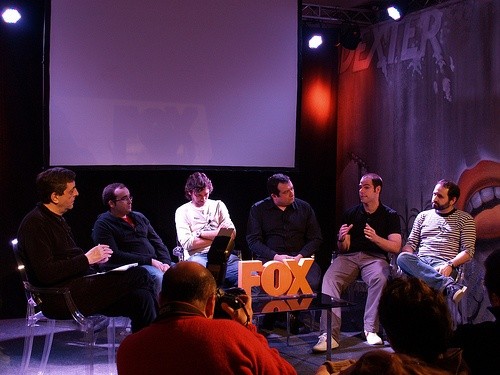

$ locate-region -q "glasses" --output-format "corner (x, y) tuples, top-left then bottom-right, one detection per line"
(113, 197), (132, 202)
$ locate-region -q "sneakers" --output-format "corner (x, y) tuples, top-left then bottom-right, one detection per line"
(364, 330), (382, 345)
(312, 332), (339, 351)
(446, 282), (467, 303)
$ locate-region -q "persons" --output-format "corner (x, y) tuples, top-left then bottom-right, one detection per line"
(18, 168), (159, 334)
(173, 173), (263, 309)
(316, 276), (474, 375)
(117, 261), (297, 375)
(89, 183), (174, 272)
(456, 251), (500, 375)
(314, 174), (401, 352)
(396, 180), (477, 326)
(244, 175), (318, 333)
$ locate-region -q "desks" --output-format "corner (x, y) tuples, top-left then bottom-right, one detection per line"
(240, 292), (351, 362)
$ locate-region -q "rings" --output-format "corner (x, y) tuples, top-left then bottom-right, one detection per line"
(103, 247), (106, 251)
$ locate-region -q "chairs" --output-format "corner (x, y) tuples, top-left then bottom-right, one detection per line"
(11, 229), (465, 375)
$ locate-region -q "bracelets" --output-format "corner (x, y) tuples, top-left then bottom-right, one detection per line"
(337, 238), (345, 243)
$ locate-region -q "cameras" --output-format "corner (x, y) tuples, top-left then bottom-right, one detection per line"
(213, 286), (247, 321)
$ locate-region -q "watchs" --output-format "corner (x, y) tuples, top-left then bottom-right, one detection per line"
(448, 261), (454, 269)
